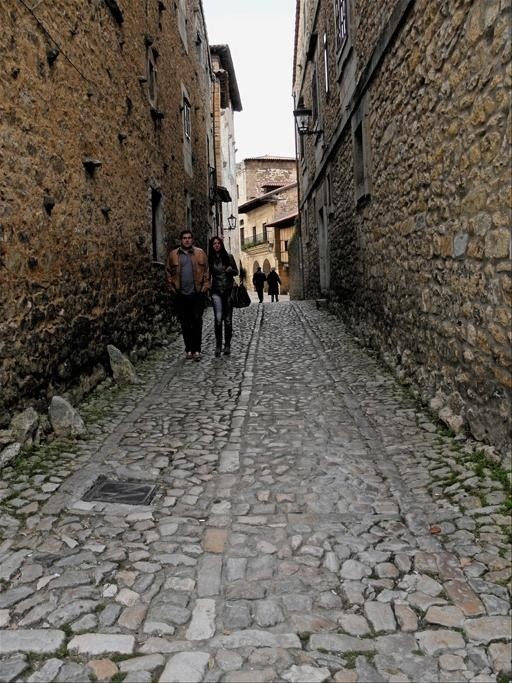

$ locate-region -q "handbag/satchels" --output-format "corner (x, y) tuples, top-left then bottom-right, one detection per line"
(231, 284), (250, 308)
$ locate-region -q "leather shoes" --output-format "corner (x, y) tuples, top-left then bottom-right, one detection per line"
(187, 351), (200, 361)
(215, 346), (230, 356)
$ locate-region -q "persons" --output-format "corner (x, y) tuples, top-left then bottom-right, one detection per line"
(205, 235), (239, 359)
(164, 228), (209, 362)
(267, 267), (283, 303)
(252, 265), (267, 303)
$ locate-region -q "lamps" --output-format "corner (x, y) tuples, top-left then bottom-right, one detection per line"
(290, 95), (325, 138)
(224, 212), (241, 231)
